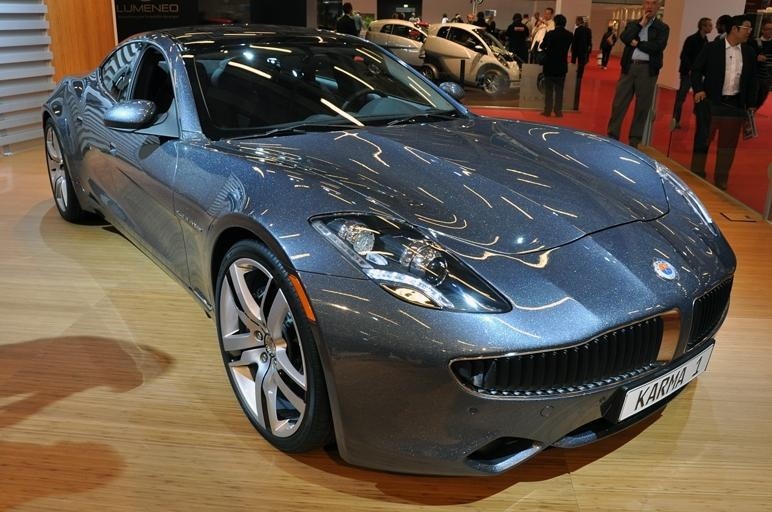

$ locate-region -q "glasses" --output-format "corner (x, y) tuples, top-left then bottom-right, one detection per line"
(738, 26), (752, 31)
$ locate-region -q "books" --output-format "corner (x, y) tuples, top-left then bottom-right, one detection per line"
(741, 108), (759, 144)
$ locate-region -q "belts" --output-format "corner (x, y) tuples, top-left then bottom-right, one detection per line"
(631, 60), (648, 64)
(723, 95), (736, 100)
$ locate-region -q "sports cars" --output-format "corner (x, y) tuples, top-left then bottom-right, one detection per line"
(42, 27), (737, 476)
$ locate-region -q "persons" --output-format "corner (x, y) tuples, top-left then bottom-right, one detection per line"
(576, 15), (584, 26)
(689, 15), (758, 189)
(712, 15), (732, 42)
(672, 17), (714, 127)
(540, 13), (574, 117)
(336, 1), (556, 64)
(606, 0), (670, 146)
(753, 19), (772, 114)
(597, 25), (616, 68)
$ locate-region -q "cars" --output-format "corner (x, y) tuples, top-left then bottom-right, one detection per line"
(206, 10), (236, 26)
(363, 19), (429, 75)
(491, 28), (532, 53)
(408, 20), (429, 38)
(419, 23), (526, 95)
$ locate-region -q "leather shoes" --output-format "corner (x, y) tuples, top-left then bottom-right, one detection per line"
(715, 179), (727, 189)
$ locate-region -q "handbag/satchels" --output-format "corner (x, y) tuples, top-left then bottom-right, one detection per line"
(607, 33), (614, 46)
